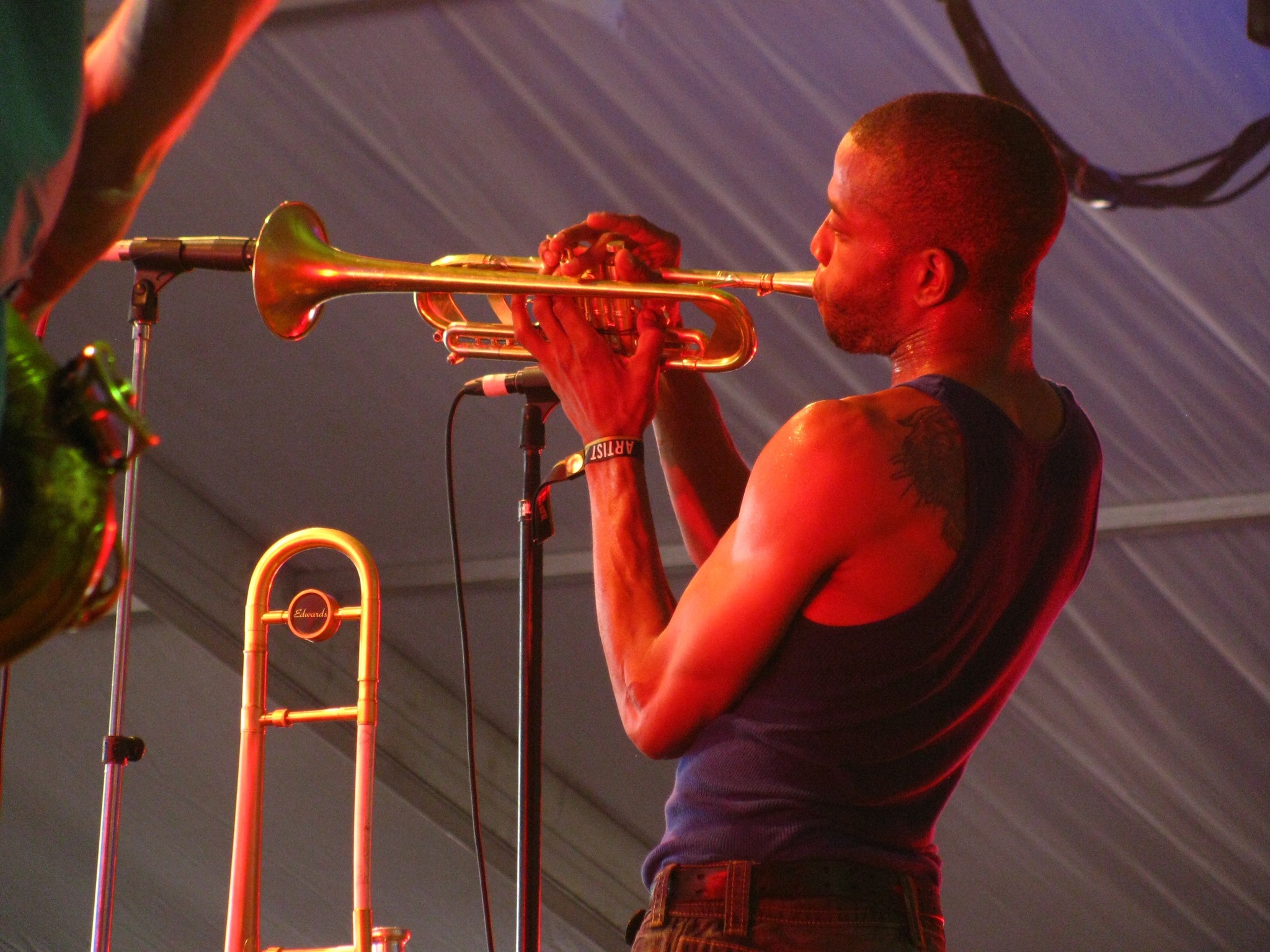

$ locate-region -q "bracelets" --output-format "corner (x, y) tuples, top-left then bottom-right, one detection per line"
(583, 435), (645, 466)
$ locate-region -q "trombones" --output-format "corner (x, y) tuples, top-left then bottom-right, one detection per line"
(224, 528), (410, 952)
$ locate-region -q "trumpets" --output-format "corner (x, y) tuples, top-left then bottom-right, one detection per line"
(252, 201), (819, 371)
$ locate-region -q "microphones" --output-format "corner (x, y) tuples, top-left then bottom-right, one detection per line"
(98, 236), (258, 273)
(465, 365), (561, 399)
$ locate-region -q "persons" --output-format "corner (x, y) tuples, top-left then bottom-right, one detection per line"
(1, 0), (287, 655)
(512, 91), (1104, 952)
